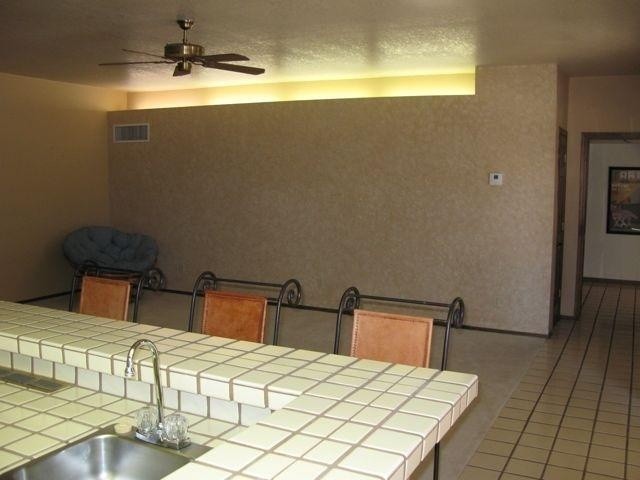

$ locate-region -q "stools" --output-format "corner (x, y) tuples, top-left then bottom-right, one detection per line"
(186, 268), (305, 346)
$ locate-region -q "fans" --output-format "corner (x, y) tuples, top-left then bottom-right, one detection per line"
(96, 16), (266, 79)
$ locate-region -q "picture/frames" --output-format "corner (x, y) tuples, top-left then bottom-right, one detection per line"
(605, 164), (640, 236)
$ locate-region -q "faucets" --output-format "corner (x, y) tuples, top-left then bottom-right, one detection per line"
(124, 339), (191, 450)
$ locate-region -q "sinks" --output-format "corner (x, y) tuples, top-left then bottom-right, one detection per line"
(0, 423), (213, 480)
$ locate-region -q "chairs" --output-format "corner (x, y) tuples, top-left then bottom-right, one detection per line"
(333, 283), (467, 479)
(67, 258), (167, 323)
(61, 223), (160, 304)
